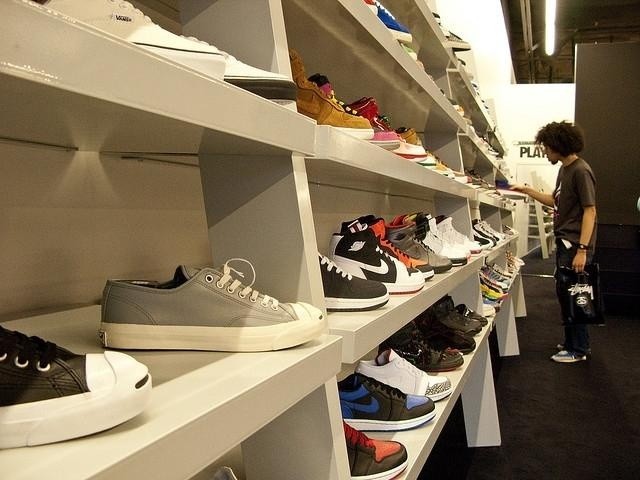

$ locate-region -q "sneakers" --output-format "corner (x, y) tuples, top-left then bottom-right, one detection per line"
(337, 297), (487, 479)
(465, 168), (497, 194)
(290, 48), (449, 174)
(98, 256), (320, 352)
(497, 179), (526, 200)
(551, 350), (587, 363)
(321, 211), (509, 310)
(0, 327), (154, 449)
(51, 0), (298, 105)
(432, 10), (471, 50)
(368, 0), (413, 42)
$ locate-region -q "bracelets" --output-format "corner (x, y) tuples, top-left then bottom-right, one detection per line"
(578, 243), (588, 250)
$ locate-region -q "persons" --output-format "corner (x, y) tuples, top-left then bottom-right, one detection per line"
(508, 120), (599, 363)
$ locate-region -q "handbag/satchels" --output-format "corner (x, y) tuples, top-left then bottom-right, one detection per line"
(555, 263), (605, 325)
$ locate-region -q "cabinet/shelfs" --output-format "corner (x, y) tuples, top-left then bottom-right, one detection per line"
(510, 172), (557, 259)
(587, 223), (639, 319)
(1, 1), (530, 480)
